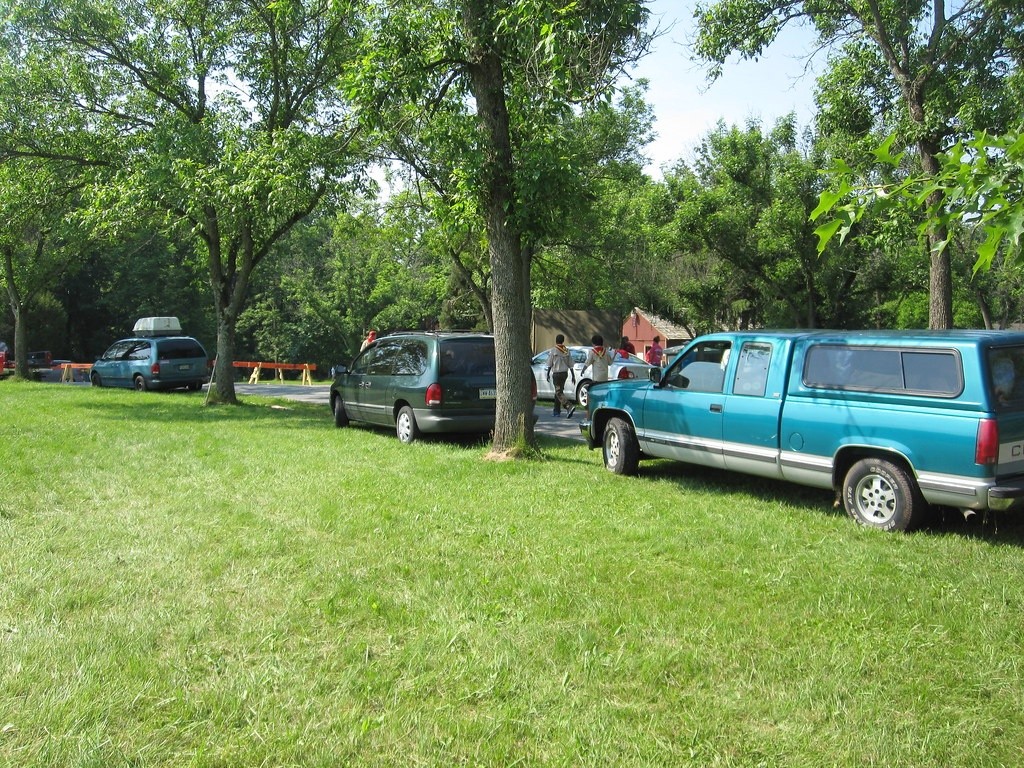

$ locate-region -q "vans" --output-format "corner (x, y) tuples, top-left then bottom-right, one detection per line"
(8, 352), (53, 368)
(88, 336), (210, 393)
(329, 329), (538, 444)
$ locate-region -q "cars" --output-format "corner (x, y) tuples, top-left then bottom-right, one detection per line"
(531, 346), (662, 409)
(33, 360), (73, 381)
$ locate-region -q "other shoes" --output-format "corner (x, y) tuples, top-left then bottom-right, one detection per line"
(552, 414), (560, 417)
(567, 407), (575, 418)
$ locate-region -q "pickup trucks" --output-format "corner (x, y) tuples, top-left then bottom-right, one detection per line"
(578, 327), (1024, 533)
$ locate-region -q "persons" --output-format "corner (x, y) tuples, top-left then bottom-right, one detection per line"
(681, 341), (696, 369)
(580, 335), (617, 385)
(619, 336), (635, 355)
(649, 336), (663, 367)
(360, 331), (377, 363)
(546, 334), (576, 419)
(331, 367), (335, 382)
